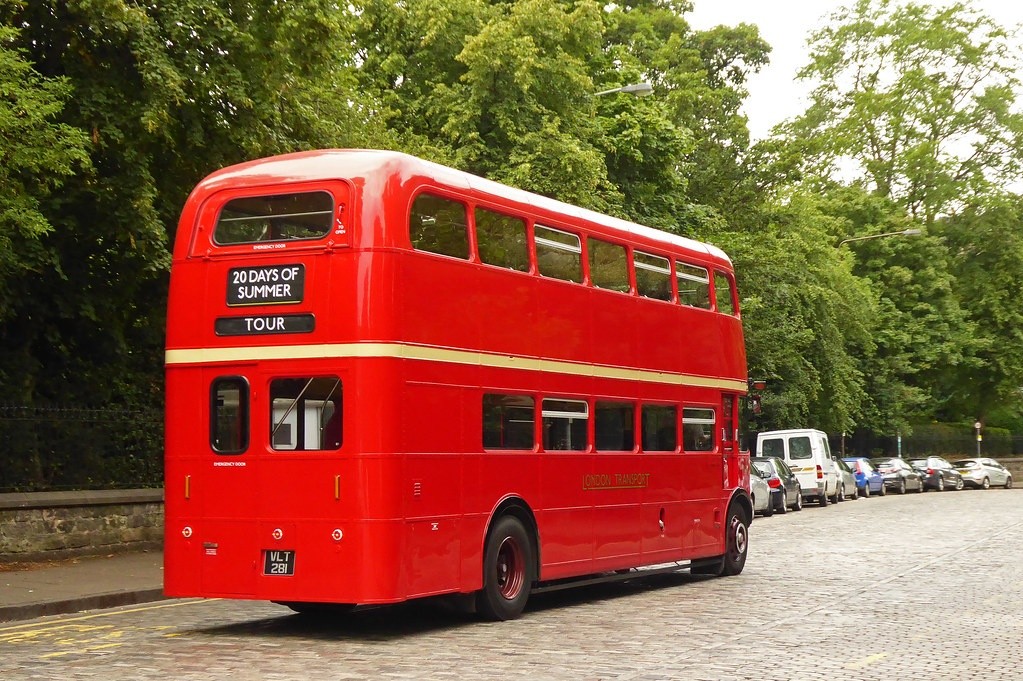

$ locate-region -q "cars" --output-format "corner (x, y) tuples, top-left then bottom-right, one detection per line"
(906, 456), (964, 492)
(750, 456), (924, 519)
(949, 458), (1012, 489)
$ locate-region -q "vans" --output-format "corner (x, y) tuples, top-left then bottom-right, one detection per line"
(756, 428), (839, 506)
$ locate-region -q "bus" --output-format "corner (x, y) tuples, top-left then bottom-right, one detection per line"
(163, 149), (755, 630)
